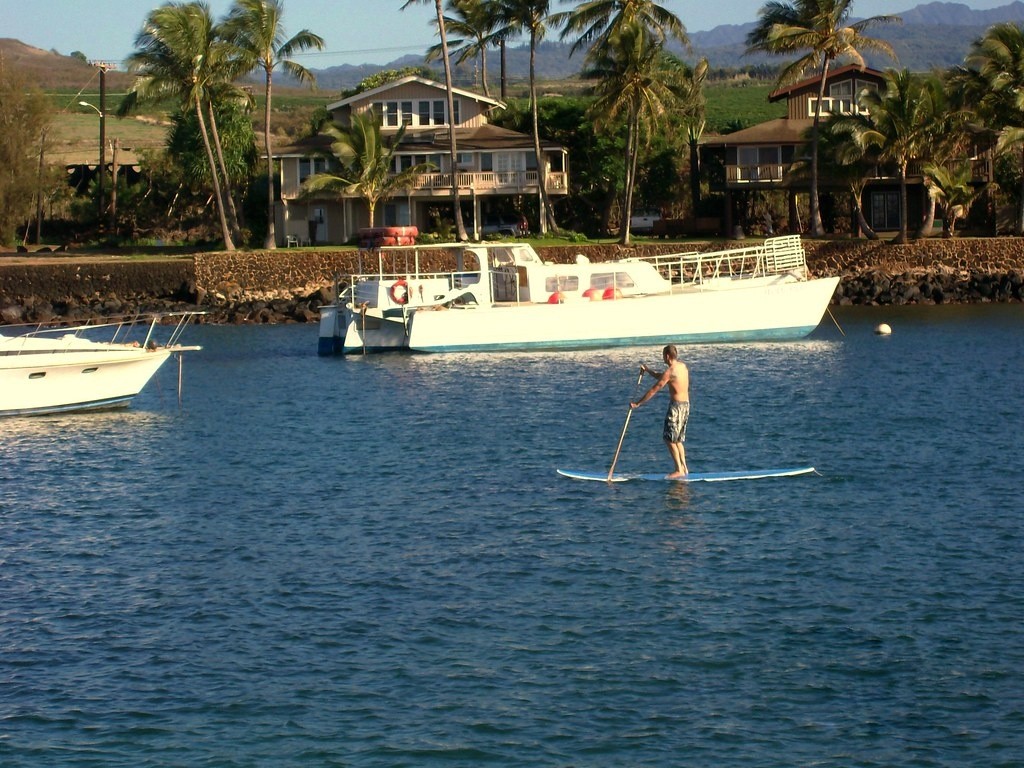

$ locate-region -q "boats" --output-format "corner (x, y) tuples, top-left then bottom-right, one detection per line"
(0, 310), (211, 420)
(316, 233), (840, 360)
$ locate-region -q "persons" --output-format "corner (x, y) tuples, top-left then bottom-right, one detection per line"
(630, 345), (690, 477)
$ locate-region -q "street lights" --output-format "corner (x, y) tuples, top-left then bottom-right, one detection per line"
(79, 101), (105, 241)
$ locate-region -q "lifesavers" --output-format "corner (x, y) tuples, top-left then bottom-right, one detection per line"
(358, 226), (419, 246)
(390, 280), (412, 304)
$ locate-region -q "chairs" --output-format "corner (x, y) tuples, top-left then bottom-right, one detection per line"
(299, 234), (311, 247)
(286, 235), (298, 249)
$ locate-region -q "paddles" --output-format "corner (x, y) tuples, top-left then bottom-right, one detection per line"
(606, 366), (645, 485)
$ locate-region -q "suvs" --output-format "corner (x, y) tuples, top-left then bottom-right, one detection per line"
(463, 211), (530, 237)
(630, 207), (666, 240)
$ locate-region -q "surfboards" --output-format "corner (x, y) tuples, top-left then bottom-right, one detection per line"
(557, 466), (816, 482)
